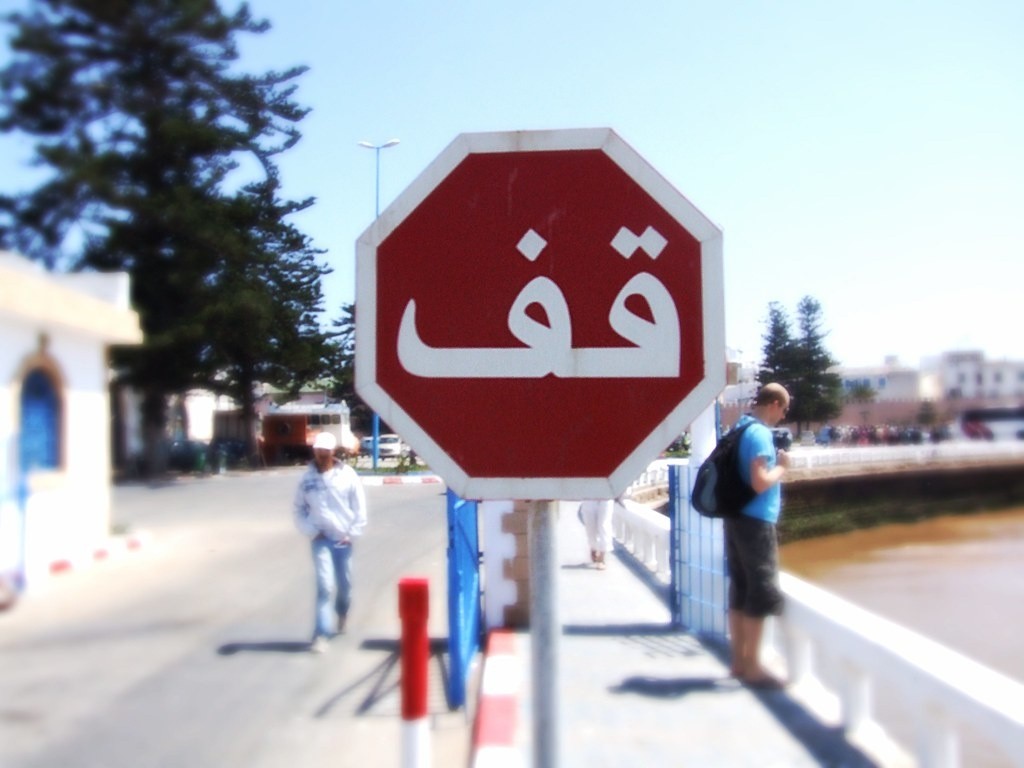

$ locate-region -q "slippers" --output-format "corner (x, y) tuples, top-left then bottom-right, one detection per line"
(728, 671), (747, 680)
(742, 677), (785, 690)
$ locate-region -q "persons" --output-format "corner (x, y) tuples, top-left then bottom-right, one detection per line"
(723, 380), (793, 688)
(292, 429), (368, 656)
(775, 421), (953, 454)
(579, 497), (615, 570)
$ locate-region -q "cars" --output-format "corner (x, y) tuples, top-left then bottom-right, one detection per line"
(361, 435), (375, 458)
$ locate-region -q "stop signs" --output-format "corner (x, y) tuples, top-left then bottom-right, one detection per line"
(356, 127), (726, 503)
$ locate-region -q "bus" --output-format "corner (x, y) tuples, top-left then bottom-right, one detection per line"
(957, 406), (1024, 444)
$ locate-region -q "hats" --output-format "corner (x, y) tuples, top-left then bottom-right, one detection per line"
(312, 431), (337, 450)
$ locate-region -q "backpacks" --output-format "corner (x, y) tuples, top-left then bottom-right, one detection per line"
(692, 420), (759, 519)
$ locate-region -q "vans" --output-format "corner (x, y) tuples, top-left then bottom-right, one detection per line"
(378, 433), (412, 460)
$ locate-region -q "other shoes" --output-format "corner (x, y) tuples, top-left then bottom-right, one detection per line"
(591, 562), (599, 568)
(311, 632), (330, 653)
(337, 614), (348, 633)
(598, 562), (606, 570)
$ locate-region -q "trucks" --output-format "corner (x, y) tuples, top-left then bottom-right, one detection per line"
(213, 405), (276, 464)
(263, 411), (358, 463)
